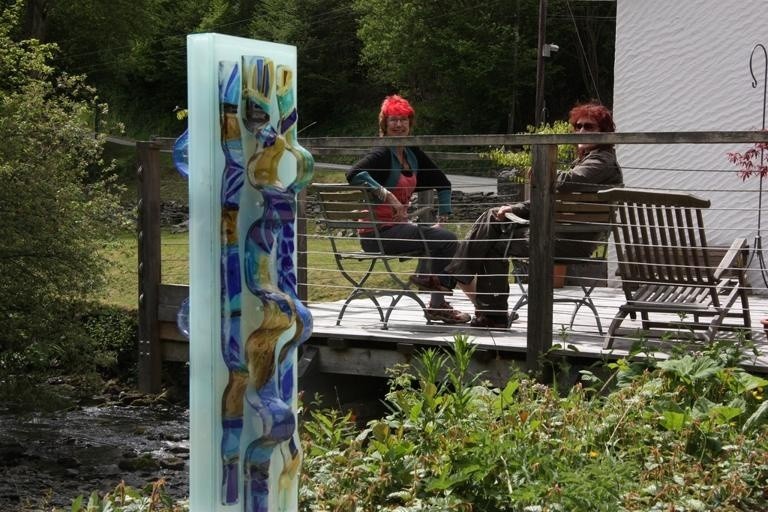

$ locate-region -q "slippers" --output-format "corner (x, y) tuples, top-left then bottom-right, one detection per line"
(409, 273), (454, 295)
(471, 316), (510, 329)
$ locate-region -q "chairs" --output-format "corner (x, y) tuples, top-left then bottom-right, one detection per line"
(311, 182), (433, 331)
(505, 179), (624, 333)
(599, 183), (752, 350)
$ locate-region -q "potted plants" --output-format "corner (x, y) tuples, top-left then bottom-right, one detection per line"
(477, 119), (582, 286)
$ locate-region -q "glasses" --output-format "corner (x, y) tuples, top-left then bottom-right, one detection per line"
(574, 121), (599, 130)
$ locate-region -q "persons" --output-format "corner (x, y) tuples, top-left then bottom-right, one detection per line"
(401, 96), (626, 331)
(343, 93), (520, 326)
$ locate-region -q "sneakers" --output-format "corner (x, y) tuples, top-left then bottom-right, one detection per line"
(427, 301), (471, 323)
